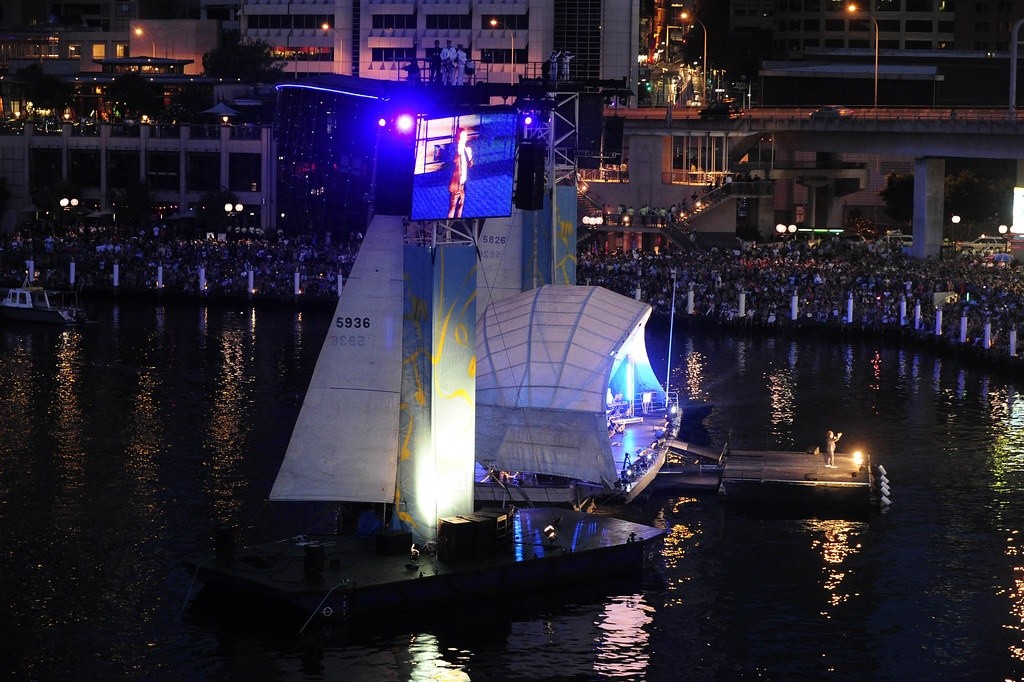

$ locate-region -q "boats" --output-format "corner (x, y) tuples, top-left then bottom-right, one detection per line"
(188, 211), (667, 634)
(0, 257), (87, 324)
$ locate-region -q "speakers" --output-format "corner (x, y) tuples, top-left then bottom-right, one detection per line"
(515, 143), (545, 210)
(602, 116), (624, 164)
(554, 93), (576, 165)
(577, 92), (604, 169)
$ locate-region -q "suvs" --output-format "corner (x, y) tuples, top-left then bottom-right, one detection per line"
(961, 236), (1012, 254)
(876, 235), (913, 247)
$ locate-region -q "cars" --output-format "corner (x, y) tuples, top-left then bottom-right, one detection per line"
(842, 234), (875, 246)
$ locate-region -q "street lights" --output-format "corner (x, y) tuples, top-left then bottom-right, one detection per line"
(582, 215), (603, 253)
(681, 13), (707, 103)
(999, 224), (1015, 255)
(491, 20), (513, 86)
(225, 202), (244, 248)
(135, 28), (155, 56)
(850, 5), (878, 107)
(60, 198), (79, 240)
(776, 224), (797, 248)
(952, 216), (960, 254)
(322, 24), (342, 73)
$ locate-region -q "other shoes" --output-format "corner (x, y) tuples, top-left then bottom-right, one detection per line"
(825, 465), (838, 468)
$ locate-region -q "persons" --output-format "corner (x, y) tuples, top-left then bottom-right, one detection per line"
(824, 431), (842, 468)
(549, 51), (575, 80)
(448, 127), (473, 218)
(428, 39), (467, 86)
(0, 161), (1024, 346)
(606, 387), (616, 404)
